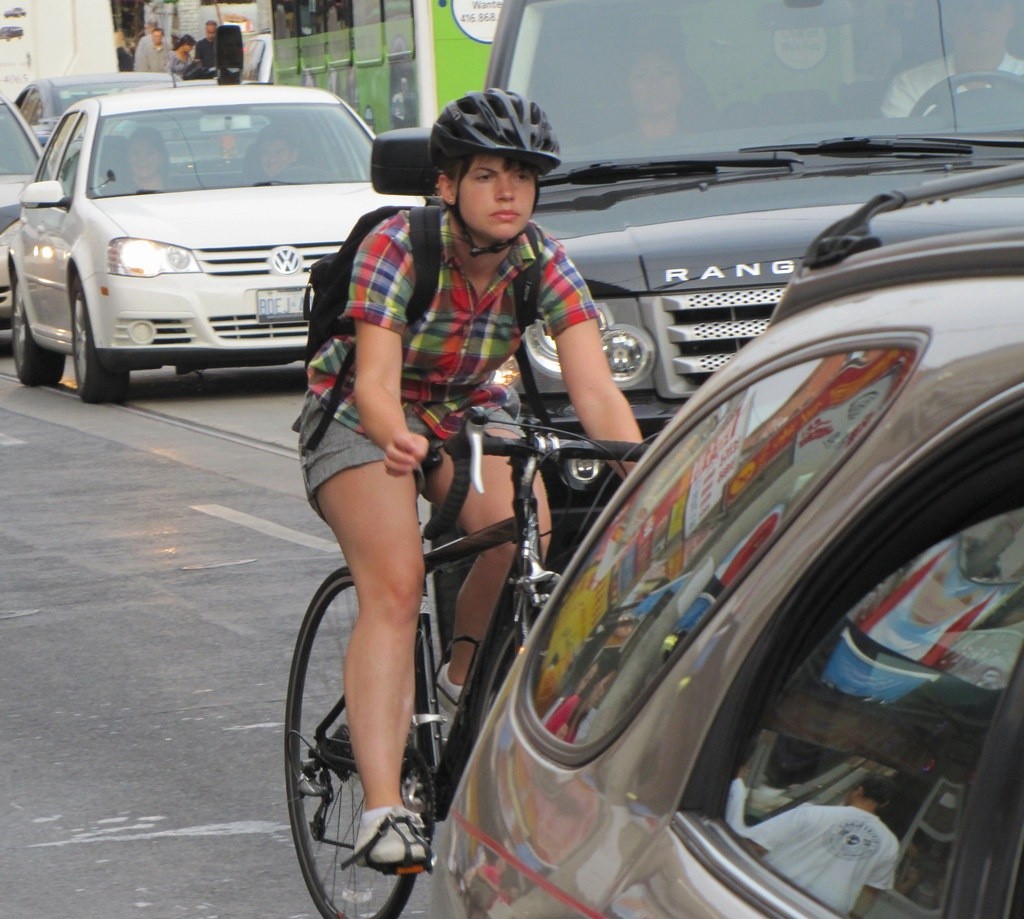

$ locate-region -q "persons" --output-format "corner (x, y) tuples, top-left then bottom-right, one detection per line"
(883, 0), (1024, 121)
(725, 728), (902, 918)
(239, 124), (305, 185)
(542, 647), (620, 742)
(593, 46), (687, 153)
(291, 88), (649, 865)
(115, 14), (218, 72)
(99, 126), (181, 196)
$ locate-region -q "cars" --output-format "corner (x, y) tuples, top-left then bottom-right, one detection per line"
(9, 72), (185, 158)
(8, 85), (429, 407)
(235, 28), (272, 83)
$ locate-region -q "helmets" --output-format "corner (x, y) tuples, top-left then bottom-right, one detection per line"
(426, 87), (561, 177)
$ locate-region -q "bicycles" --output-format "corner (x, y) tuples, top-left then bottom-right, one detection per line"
(285, 406), (660, 919)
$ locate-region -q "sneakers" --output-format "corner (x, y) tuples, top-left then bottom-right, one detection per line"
(435, 662), (465, 721)
(353, 806), (429, 867)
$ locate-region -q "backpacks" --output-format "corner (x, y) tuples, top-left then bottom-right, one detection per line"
(303, 206), (541, 377)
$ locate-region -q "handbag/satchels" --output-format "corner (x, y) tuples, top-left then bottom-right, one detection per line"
(182, 63), (217, 79)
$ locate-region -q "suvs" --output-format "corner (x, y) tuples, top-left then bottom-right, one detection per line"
(371, 2), (1023, 580)
(420, 162), (1024, 919)
(1, 98), (54, 332)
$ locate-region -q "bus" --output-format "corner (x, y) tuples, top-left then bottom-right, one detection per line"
(271, 0), (513, 137)
